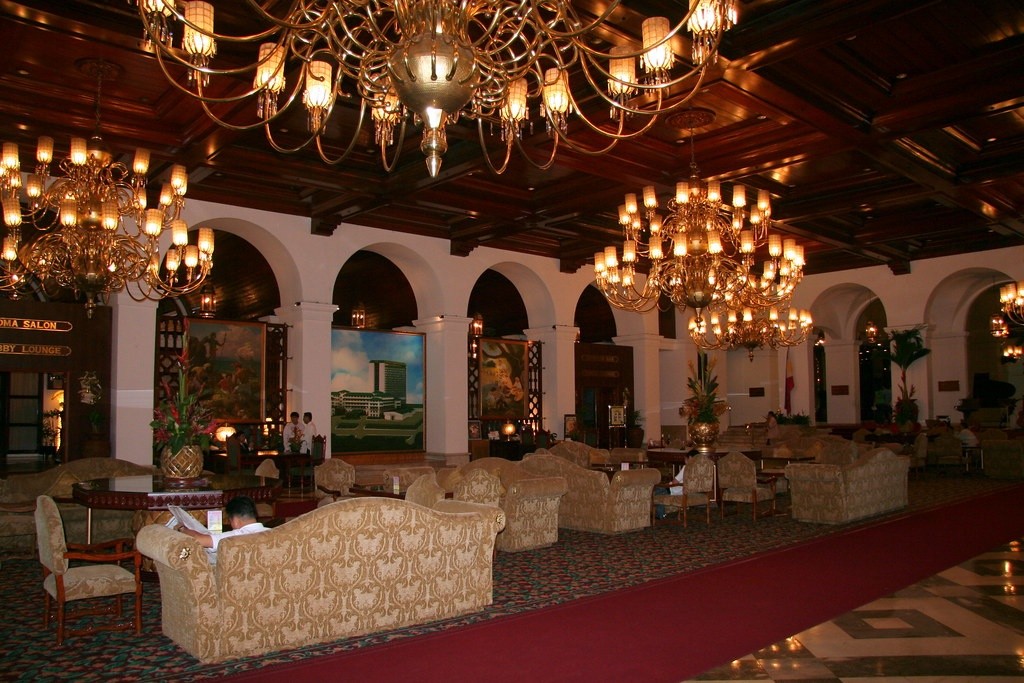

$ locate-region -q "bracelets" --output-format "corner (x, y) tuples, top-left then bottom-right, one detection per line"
(178, 526), (185, 532)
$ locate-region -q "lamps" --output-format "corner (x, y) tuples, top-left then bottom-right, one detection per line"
(502, 416), (516, 443)
(2, 0), (1023, 366)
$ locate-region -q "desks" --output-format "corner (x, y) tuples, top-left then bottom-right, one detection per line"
(648, 445), (761, 509)
(216, 451), (308, 486)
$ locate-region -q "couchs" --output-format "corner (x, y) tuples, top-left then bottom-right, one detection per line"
(0, 400), (1023, 663)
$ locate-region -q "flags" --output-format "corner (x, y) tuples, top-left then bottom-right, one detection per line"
(783, 352), (795, 415)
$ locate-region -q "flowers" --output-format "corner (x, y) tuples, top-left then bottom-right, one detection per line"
(288, 424), (305, 444)
(149, 314), (223, 453)
(678, 350), (728, 424)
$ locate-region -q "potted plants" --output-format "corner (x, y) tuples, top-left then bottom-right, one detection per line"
(626, 410), (644, 449)
(884, 323), (932, 423)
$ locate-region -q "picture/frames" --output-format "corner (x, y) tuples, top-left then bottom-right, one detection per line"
(184, 317), (266, 424)
(477, 337), (530, 422)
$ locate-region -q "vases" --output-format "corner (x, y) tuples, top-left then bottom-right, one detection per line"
(688, 419), (720, 453)
(291, 443), (303, 453)
(161, 440), (204, 478)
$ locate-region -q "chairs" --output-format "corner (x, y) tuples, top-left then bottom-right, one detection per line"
(650, 454), (715, 528)
(227, 437), (261, 487)
(33, 495), (141, 646)
(714, 450), (776, 523)
(301, 434), (326, 489)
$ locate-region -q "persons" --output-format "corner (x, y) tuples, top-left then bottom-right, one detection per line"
(283, 412), (305, 453)
(235, 431), (249, 454)
(766, 411), (780, 446)
(656, 449), (699, 520)
(300, 412), (317, 455)
(957, 421), (978, 448)
(175, 495), (272, 552)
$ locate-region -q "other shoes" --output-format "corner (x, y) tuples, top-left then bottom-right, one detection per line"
(651, 512), (667, 519)
